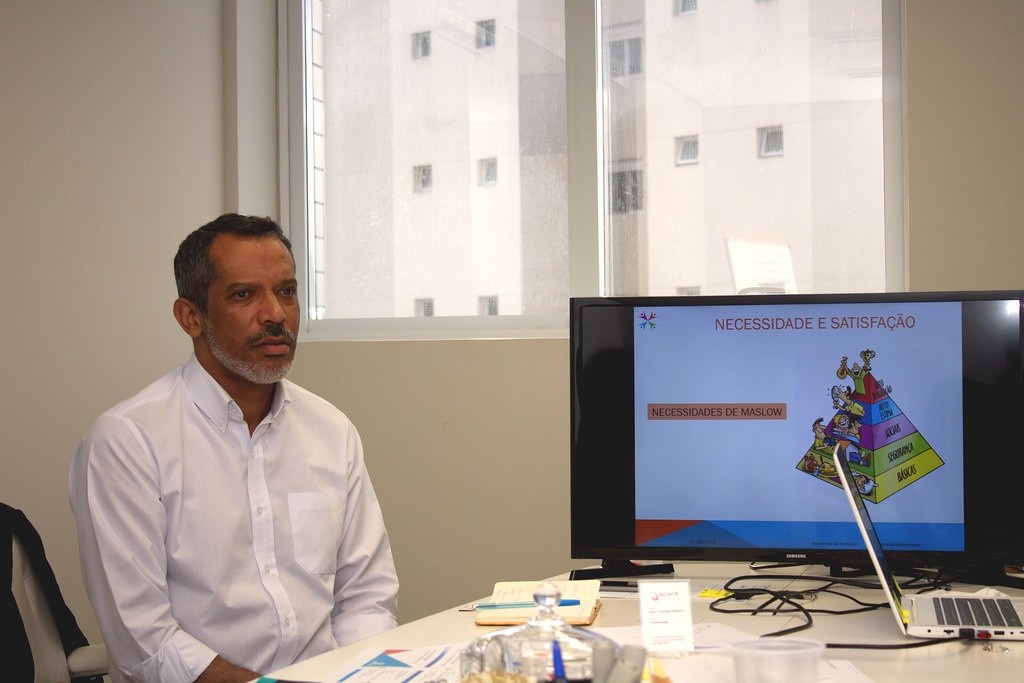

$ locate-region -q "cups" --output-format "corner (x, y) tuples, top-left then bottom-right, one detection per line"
(730, 639), (826, 683)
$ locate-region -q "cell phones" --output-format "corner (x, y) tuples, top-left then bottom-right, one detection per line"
(599, 581), (638, 592)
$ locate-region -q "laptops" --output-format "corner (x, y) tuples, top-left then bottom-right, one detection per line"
(833, 443), (1024, 641)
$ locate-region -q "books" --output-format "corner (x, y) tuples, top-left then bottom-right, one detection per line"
(476, 579), (603, 625)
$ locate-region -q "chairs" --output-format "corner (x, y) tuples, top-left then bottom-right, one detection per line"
(0, 502), (335, 683)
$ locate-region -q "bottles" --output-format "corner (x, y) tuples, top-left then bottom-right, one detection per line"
(460, 582), (646, 683)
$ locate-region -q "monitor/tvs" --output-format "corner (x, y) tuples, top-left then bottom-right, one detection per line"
(570, 289), (1024, 585)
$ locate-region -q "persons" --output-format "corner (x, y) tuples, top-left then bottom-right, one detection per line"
(69, 212), (399, 683)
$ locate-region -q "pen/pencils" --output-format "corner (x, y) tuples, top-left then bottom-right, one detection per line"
(473, 599), (581, 610)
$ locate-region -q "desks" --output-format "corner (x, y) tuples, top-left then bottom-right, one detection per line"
(248, 548), (1024, 682)
